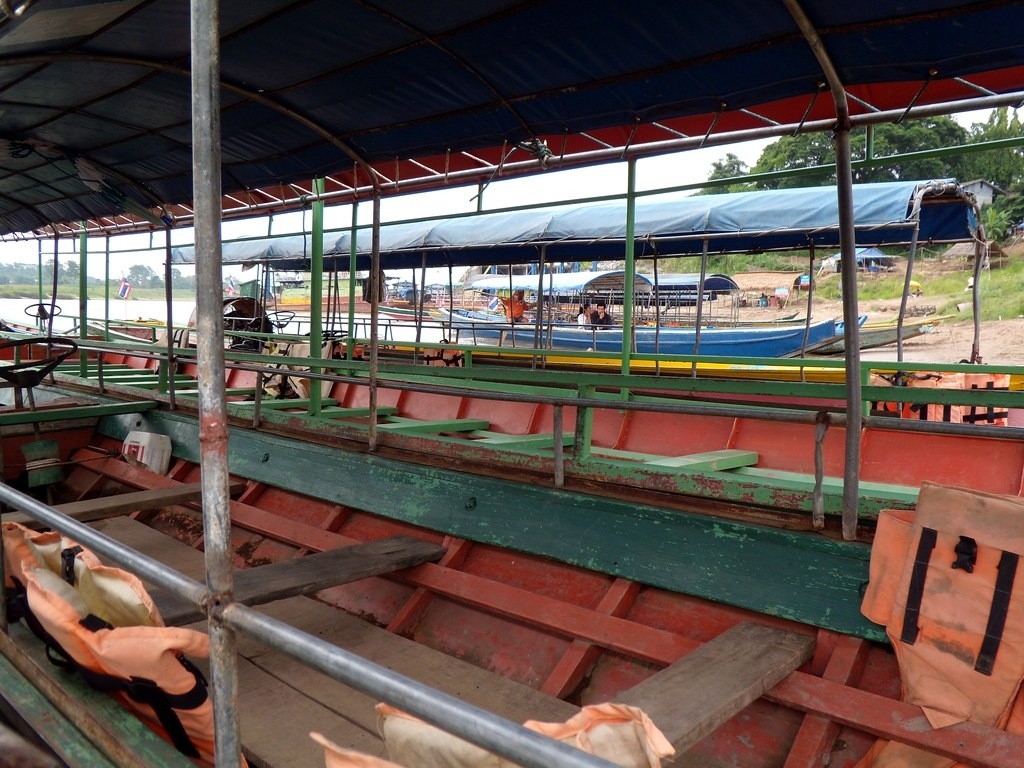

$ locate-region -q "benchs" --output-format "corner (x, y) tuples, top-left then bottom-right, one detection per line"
(471, 431), (575, 450)
(376, 417), (490, 434)
(289, 405), (398, 418)
(606, 620), (815, 765)
(35, 363), (265, 397)
(147, 532), (447, 627)
(227, 396), (339, 410)
(644, 449), (758, 471)
(1, 479), (246, 531)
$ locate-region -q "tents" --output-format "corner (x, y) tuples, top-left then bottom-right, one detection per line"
(830, 248), (889, 274)
(723, 271), (817, 304)
(942, 240), (1008, 270)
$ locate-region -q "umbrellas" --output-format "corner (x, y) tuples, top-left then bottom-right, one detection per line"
(901, 280), (921, 288)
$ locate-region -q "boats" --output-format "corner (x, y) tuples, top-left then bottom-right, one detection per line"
(428, 303), (956, 357)
(438, 304), (834, 361)
(377, 305), (434, 323)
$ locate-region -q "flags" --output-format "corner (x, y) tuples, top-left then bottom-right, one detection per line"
(489, 293), (498, 310)
(118, 270), (131, 299)
(227, 277), (234, 294)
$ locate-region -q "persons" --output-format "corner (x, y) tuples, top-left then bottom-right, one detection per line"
(496, 291), (537, 323)
(907, 289), (921, 297)
(577, 303), (592, 330)
(590, 302), (612, 330)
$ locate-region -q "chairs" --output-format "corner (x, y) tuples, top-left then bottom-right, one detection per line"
(767, 297), (779, 306)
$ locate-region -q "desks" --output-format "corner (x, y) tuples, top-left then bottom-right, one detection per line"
(752, 298), (762, 307)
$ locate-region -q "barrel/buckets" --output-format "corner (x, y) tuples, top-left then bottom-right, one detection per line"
(123, 430), (172, 476)
